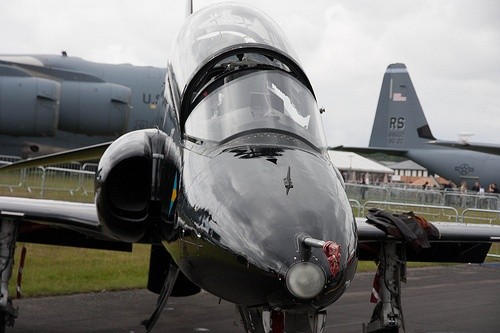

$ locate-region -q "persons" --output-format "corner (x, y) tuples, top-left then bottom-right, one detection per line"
(360, 174), (498, 211)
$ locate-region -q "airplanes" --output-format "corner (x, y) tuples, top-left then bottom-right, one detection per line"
(0, 1), (500, 333)
